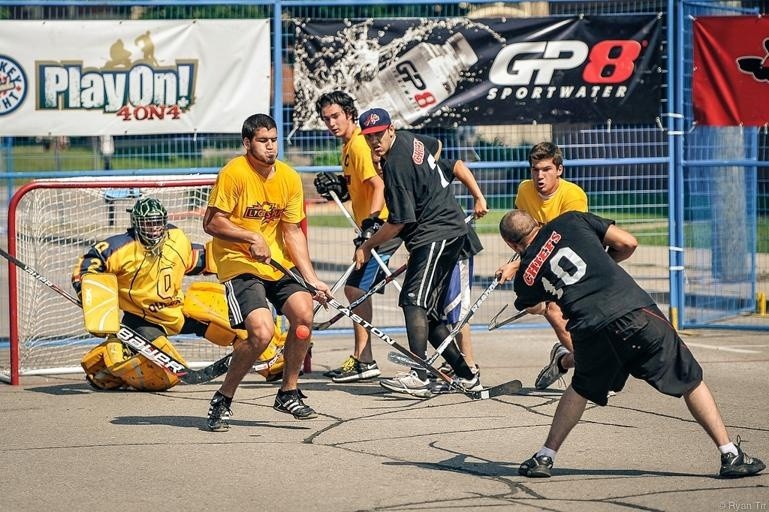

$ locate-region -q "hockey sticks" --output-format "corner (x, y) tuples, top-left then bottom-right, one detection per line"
(0, 251), (233, 384)
(249, 246), (522, 400)
(226, 257), (356, 373)
(488, 303), (529, 331)
(314, 205), (490, 331)
(387, 252), (520, 371)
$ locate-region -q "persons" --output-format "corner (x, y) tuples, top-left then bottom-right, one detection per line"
(316, 92), (406, 383)
(203, 114), (335, 432)
(494, 142), (590, 392)
(73, 197), (306, 392)
(371, 149), (490, 381)
(353, 108), (483, 398)
(499, 209), (767, 478)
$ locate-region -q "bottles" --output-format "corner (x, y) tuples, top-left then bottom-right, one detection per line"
(356, 31), (478, 131)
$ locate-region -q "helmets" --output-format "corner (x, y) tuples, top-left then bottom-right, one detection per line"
(130, 199), (168, 260)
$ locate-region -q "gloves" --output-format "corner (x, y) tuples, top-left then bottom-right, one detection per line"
(313, 171), (350, 203)
(354, 218), (385, 258)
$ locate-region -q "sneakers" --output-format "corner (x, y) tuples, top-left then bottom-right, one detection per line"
(208, 398), (235, 433)
(520, 452), (555, 478)
(323, 363), (348, 376)
(333, 356), (382, 383)
(720, 435), (767, 477)
(428, 360), (456, 380)
(273, 391), (317, 422)
(536, 342), (571, 390)
(381, 371), (433, 401)
(266, 371), (306, 387)
(439, 375), (485, 395)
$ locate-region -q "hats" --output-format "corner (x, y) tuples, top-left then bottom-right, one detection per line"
(358, 108), (392, 137)
